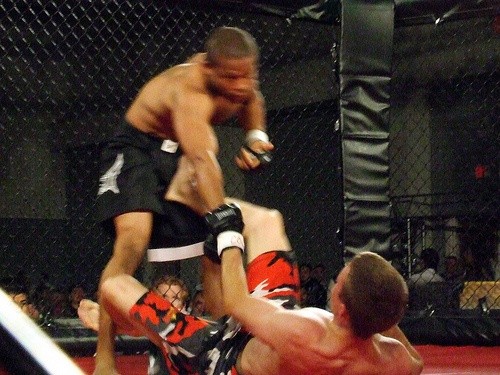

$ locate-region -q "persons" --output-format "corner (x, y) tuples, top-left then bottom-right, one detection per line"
(155, 276), (209, 315)
(392, 246), (496, 330)
(299, 263), (343, 310)
(78, 198), (424, 375)
(93, 27), (273, 375)
(0, 276), (100, 336)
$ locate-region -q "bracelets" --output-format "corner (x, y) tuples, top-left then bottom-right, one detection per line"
(217, 231), (244, 256)
(246, 130), (269, 142)
